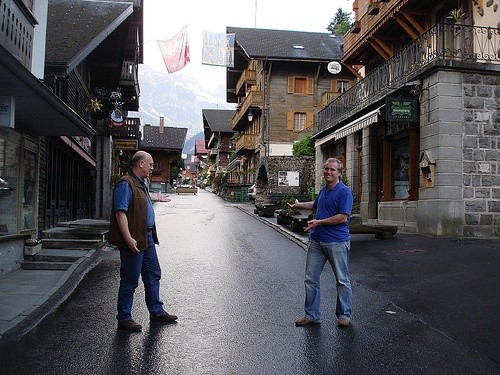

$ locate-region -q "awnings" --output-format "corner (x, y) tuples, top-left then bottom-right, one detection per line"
(335, 103), (386, 140)
(0, 44), (97, 137)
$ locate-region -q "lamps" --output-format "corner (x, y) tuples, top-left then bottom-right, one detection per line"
(247, 108), (259, 121)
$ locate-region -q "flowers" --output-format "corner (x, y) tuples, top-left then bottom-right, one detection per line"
(26, 238), (42, 246)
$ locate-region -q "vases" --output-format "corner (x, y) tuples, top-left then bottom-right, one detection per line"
(25, 243), (42, 256)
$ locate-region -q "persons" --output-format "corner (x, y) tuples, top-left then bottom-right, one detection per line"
(287, 158), (352, 326)
(107, 151), (177, 330)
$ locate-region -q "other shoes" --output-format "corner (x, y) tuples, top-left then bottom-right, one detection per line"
(337, 315), (351, 326)
(150, 311), (178, 322)
(118, 320), (142, 330)
(295, 317), (321, 325)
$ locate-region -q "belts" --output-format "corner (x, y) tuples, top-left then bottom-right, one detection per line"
(146, 228), (153, 232)
